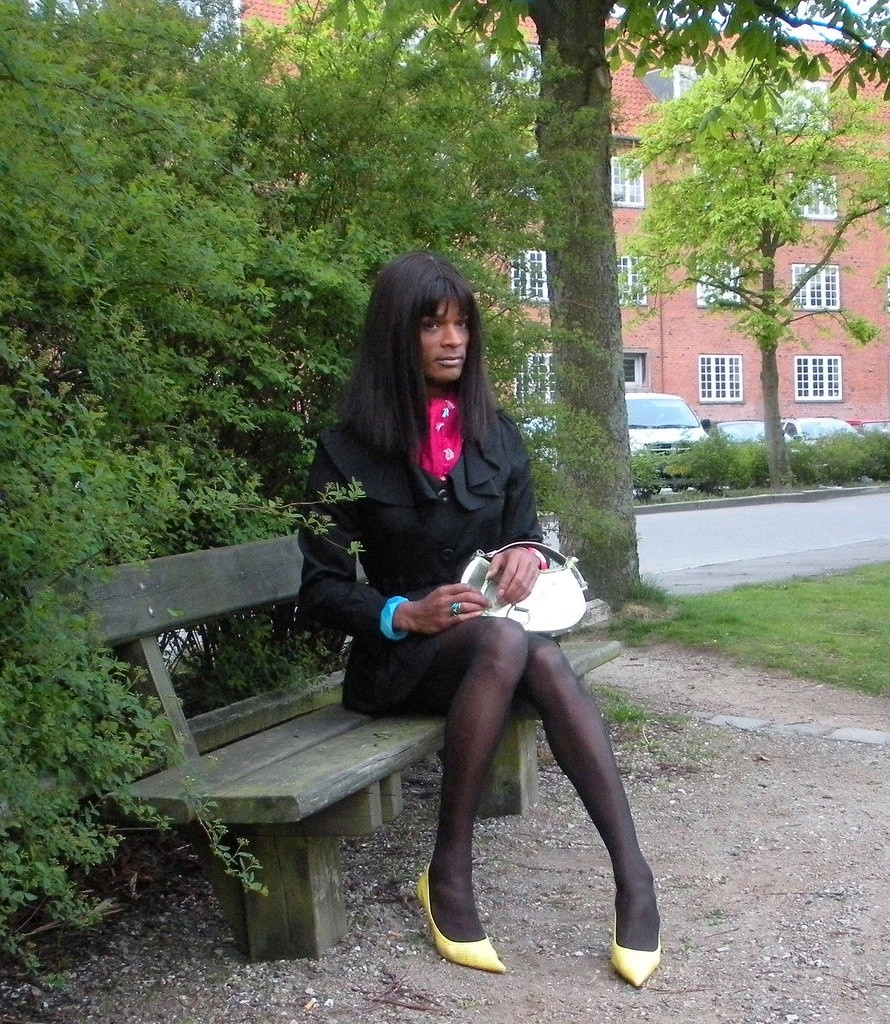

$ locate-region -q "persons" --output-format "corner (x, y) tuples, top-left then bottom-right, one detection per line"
(297, 254), (663, 988)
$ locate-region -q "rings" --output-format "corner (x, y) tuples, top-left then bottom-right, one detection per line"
(452, 602), (460, 616)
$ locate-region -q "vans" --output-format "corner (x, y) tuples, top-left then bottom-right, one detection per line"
(624, 392), (717, 495)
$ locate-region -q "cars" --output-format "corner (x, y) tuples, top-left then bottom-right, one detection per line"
(847, 419), (890, 442)
(780, 417), (865, 446)
(713, 419), (792, 445)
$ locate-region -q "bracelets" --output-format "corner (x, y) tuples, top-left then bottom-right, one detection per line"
(528, 547), (548, 569)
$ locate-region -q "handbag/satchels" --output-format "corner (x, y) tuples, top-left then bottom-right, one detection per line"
(454, 538), (588, 634)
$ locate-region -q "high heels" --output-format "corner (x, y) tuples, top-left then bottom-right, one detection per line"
(611, 890), (662, 987)
(418, 862), (507, 975)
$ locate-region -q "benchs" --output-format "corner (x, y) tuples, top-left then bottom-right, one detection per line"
(90, 531), (619, 960)
